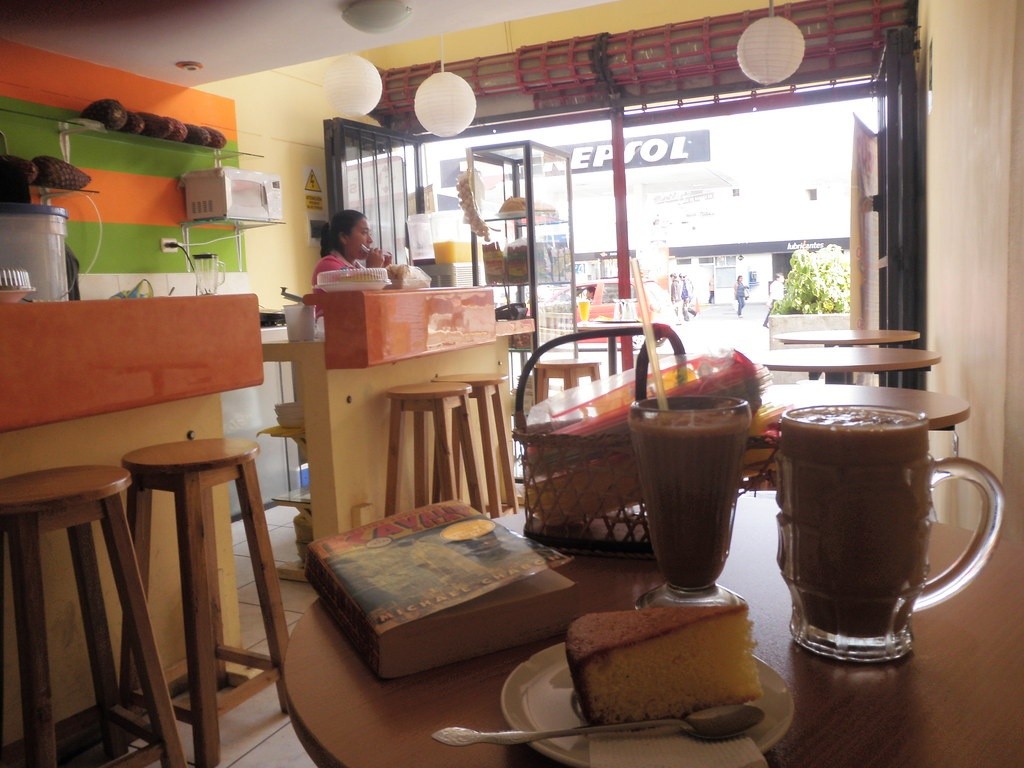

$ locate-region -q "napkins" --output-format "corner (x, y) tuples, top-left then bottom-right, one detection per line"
(586, 726), (770, 768)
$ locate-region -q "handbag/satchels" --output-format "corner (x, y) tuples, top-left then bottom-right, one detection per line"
(744, 288), (749, 298)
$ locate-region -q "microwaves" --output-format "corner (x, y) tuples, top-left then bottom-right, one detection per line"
(185, 167), (284, 222)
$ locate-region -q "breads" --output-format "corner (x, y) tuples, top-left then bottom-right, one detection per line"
(339, 273), (384, 281)
(386, 263), (412, 279)
(565, 603), (764, 727)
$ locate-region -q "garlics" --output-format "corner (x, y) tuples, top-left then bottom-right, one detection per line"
(457, 177), (491, 242)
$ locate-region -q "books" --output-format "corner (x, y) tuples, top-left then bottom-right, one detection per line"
(307, 500), (576, 679)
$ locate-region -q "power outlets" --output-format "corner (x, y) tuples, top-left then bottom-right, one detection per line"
(160, 237), (178, 253)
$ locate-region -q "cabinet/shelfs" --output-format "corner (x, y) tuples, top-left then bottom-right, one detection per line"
(0, 107), (288, 272)
(467, 140), (581, 400)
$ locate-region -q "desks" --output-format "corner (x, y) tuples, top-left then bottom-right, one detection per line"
(762, 381), (972, 433)
(773, 330), (921, 384)
(758, 346), (943, 385)
(575, 320), (643, 376)
(284, 495), (1024, 768)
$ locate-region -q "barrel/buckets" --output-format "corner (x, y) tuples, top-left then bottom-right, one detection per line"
(0, 203), (79, 303)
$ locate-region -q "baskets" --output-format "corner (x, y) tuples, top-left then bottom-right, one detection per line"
(511, 325), (687, 561)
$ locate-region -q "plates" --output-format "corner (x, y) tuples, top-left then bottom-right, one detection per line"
(0, 288), (38, 304)
(499, 639), (795, 767)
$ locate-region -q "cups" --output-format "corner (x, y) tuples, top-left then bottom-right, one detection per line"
(613, 298), (638, 321)
(628, 396), (751, 619)
(283, 303), (316, 342)
(192, 254), (226, 296)
(577, 300), (590, 324)
(775, 404), (1005, 666)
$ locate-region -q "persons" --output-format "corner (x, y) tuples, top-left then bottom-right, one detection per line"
(763, 272), (784, 328)
(709, 274), (714, 303)
(670, 272), (697, 325)
(586, 286), (596, 301)
(312, 209), (393, 323)
(734, 275), (747, 318)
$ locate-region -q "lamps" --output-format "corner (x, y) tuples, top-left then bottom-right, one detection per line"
(413, 32), (477, 140)
(323, 54), (384, 118)
(735, 0), (807, 87)
(339, 0), (413, 36)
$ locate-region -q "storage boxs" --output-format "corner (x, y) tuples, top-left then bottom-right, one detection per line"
(0, 202), (71, 302)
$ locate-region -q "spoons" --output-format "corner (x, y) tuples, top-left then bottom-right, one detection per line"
(430, 706), (766, 746)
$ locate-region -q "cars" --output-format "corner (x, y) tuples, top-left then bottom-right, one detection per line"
(526, 278), (675, 347)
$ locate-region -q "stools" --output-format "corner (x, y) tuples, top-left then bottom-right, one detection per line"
(0, 464), (188, 768)
(432, 373), (520, 520)
(535, 358), (603, 405)
(120, 437), (290, 768)
(383, 381), (488, 528)
